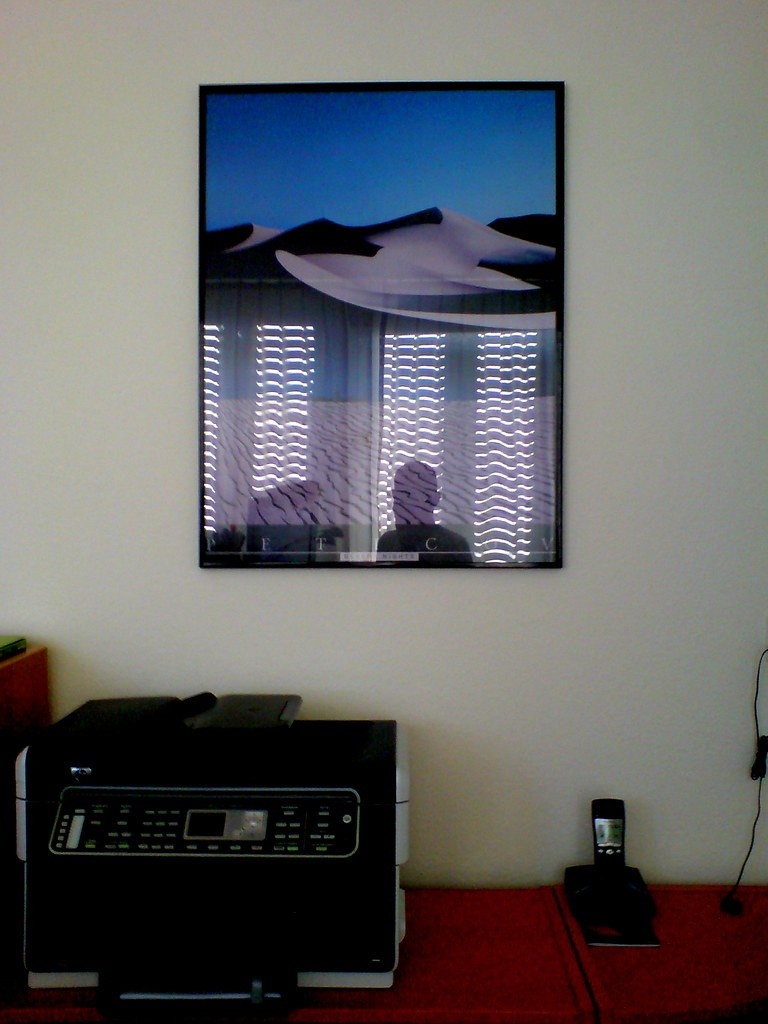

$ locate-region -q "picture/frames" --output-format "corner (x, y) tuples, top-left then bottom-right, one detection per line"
(199, 80), (566, 568)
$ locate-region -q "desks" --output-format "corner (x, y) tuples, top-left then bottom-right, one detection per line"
(0, 883), (595, 1024)
(552, 883), (768, 1024)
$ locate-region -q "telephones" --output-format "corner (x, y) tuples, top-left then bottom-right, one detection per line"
(564, 797), (658, 922)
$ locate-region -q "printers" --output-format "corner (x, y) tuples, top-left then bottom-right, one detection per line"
(16, 693), (411, 1024)
